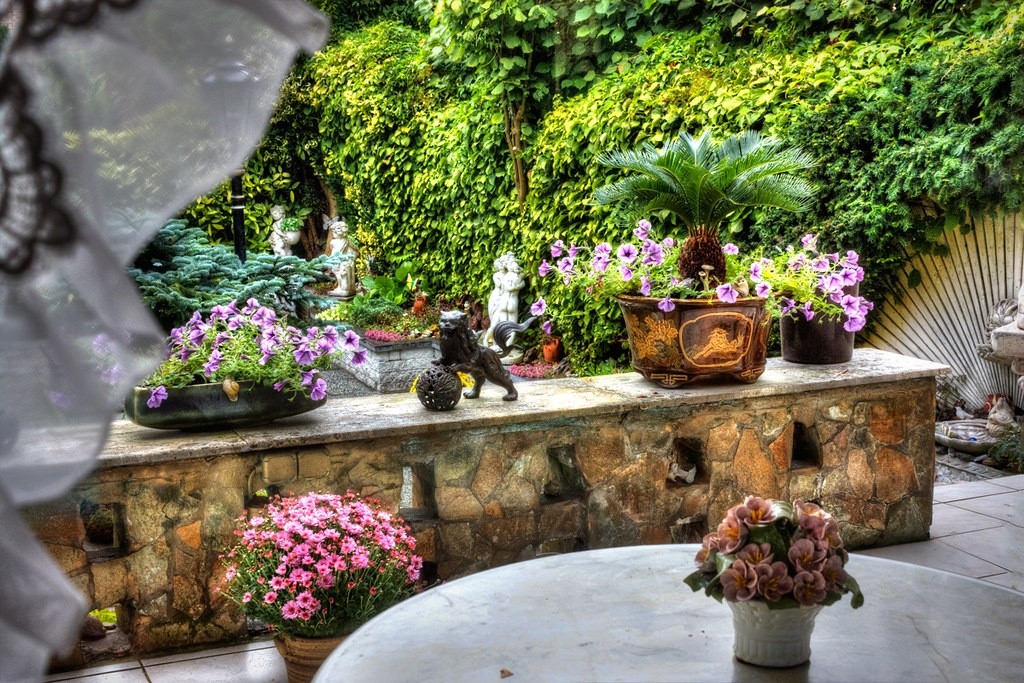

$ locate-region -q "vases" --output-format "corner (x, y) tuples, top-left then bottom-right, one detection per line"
(725, 598), (824, 667)
(123, 374), (328, 431)
(611, 291), (770, 390)
(775, 279), (861, 364)
(272, 632), (352, 683)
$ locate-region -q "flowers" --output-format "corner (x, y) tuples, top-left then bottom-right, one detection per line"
(682, 496), (865, 610)
(210, 489), (446, 643)
(533, 141), (873, 333)
(138, 296), (367, 409)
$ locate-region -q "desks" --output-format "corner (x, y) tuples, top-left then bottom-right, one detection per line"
(310, 542), (1024, 683)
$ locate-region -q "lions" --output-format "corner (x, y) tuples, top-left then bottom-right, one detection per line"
(430, 310), (538, 402)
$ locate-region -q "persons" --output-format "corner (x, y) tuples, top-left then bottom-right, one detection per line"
(324, 220), (359, 295)
(267, 206), (293, 256)
(482, 252), (527, 348)
